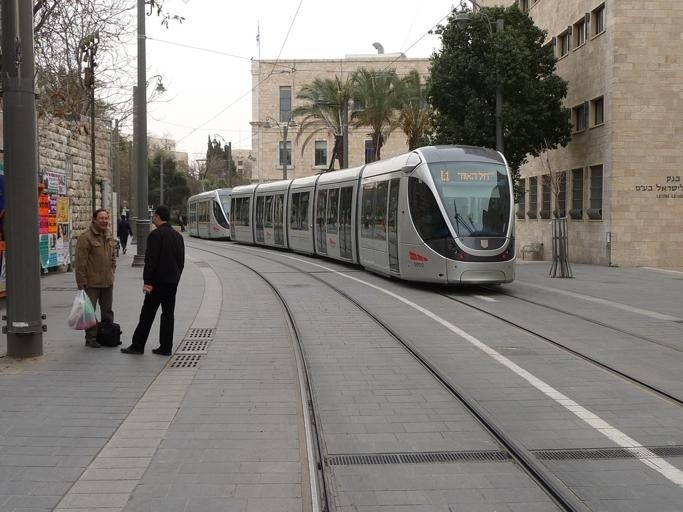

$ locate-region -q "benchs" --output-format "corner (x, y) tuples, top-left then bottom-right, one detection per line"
(522, 243), (543, 260)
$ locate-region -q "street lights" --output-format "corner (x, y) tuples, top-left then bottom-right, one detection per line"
(455, 0), (505, 158)
(261, 114), (297, 181)
(131, 74), (169, 245)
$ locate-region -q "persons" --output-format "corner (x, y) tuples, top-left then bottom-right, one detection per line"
(117, 214), (132, 254)
(120, 204), (185, 356)
(73, 208), (123, 349)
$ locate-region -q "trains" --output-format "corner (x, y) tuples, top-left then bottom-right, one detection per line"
(231, 143), (516, 284)
(185, 187), (233, 239)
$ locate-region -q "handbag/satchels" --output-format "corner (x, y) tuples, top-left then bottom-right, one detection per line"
(98, 322), (121, 347)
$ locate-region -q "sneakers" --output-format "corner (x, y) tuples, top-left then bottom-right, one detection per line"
(86, 340), (101, 348)
(152, 346), (172, 356)
(121, 345), (145, 354)
